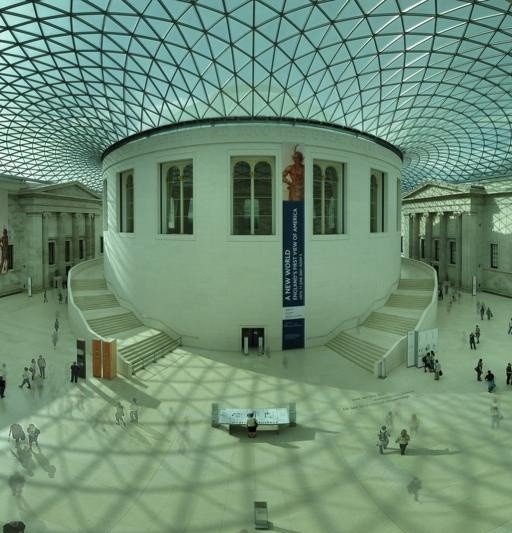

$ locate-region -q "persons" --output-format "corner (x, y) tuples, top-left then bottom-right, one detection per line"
(376, 425), (390, 454)
(485, 369), (496, 392)
(8, 422), (26, 441)
(246, 412), (258, 438)
(407, 412), (421, 439)
(407, 475), (422, 501)
(394, 428), (410, 457)
(115, 401), (126, 425)
(129, 396), (138, 422)
(505, 362), (512, 385)
(384, 410), (399, 429)
(26, 423), (41, 445)
(489, 397), (504, 427)
(282, 150), (304, 201)
(477, 358), (485, 381)
(469, 299), (512, 350)
(422, 349), (443, 380)
(0, 282), (80, 400)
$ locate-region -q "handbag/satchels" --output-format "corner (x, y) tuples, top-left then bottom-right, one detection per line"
(439, 370), (442, 376)
(474, 367), (481, 374)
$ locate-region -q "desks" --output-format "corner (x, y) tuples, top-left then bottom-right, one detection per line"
(217, 407), (291, 435)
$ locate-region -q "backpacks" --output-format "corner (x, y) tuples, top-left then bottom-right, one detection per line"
(422, 355), (427, 362)
(379, 429), (387, 442)
